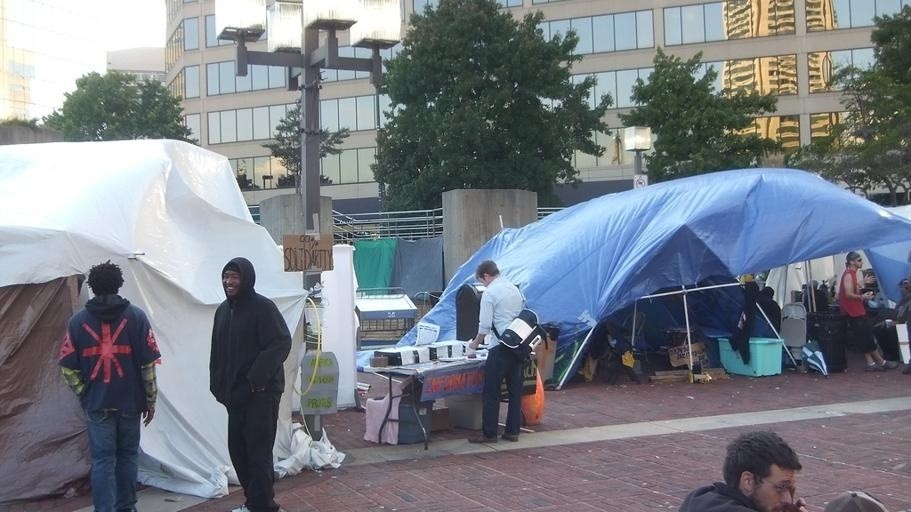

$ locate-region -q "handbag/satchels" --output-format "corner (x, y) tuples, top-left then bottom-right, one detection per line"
(499, 306), (545, 359)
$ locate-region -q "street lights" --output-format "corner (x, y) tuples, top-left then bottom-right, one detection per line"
(624, 126), (651, 175)
(214, 0), (401, 235)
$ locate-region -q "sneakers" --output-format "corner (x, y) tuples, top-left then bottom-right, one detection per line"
(469, 432), (499, 444)
(883, 360), (899, 368)
(865, 363), (887, 372)
(502, 433), (519, 442)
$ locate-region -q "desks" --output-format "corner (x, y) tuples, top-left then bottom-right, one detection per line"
(808, 307), (897, 373)
(356, 349), (537, 451)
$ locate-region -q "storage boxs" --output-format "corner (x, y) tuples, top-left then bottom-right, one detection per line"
(716, 336), (785, 378)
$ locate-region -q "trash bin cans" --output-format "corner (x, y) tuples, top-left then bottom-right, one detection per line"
(806, 311), (848, 374)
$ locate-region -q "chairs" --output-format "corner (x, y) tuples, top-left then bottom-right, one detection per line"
(777, 302), (807, 374)
(608, 312), (648, 363)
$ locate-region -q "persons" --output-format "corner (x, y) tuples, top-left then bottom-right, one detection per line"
(804, 279), (829, 313)
(59, 260), (162, 512)
(837, 249), (899, 372)
(468, 258), (530, 443)
(206, 256), (294, 511)
(872, 275), (911, 362)
(678, 428), (811, 512)
(761, 285), (782, 336)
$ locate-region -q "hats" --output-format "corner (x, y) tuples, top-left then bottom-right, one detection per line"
(826, 491), (888, 511)
